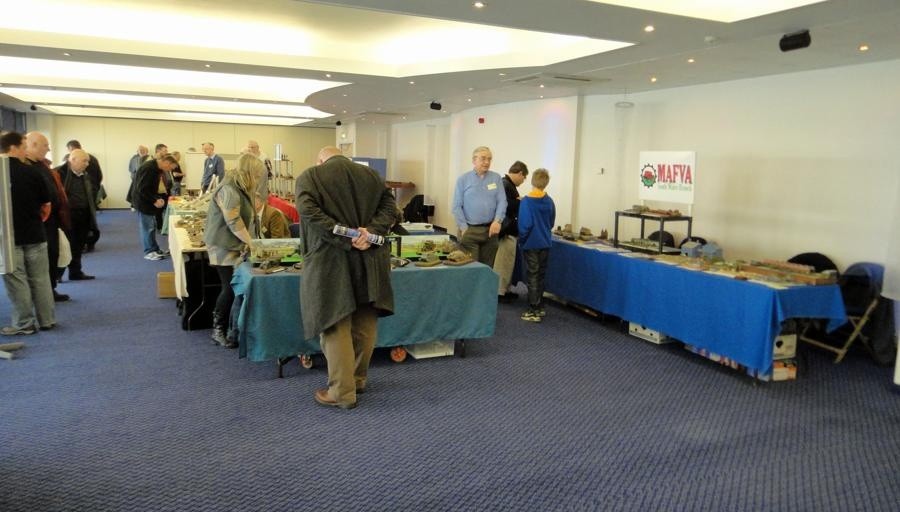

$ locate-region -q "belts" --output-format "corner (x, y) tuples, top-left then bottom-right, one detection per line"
(466, 222), (491, 227)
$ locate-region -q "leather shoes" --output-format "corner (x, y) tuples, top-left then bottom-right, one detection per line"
(312, 388), (358, 411)
(355, 383), (368, 395)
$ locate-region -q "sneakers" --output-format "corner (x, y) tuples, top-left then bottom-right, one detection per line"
(144, 251), (164, 261)
(68, 273), (95, 281)
(39, 324), (55, 331)
(0, 325), (36, 337)
(156, 249), (170, 256)
(540, 310), (547, 318)
(53, 290), (71, 303)
(521, 312), (542, 323)
(498, 292), (520, 305)
(57, 278), (63, 284)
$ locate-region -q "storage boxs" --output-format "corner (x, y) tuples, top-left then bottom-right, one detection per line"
(401, 341), (457, 360)
(775, 360), (796, 382)
(157, 272), (177, 298)
(681, 344), (738, 369)
(774, 334), (796, 360)
(629, 321), (677, 344)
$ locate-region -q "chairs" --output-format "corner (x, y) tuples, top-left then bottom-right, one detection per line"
(798, 262), (885, 369)
(786, 252), (839, 281)
(677, 237), (707, 247)
(648, 231), (677, 247)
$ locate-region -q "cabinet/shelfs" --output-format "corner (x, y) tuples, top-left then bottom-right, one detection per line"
(613, 211), (691, 255)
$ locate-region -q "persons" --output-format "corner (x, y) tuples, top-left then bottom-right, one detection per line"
(247, 140), (272, 180)
(204, 154), (264, 349)
(145, 144), (174, 230)
(0, 130), (58, 335)
(201, 142), (224, 194)
(129, 146), (150, 212)
(451, 147), (508, 269)
(54, 149), (95, 283)
(492, 161), (528, 304)
(67, 140), (103, 249)
(295, 146), (399, 409)
(255, 193), (291, 239)
(23, 130), (72, 301)
(172, 152), (186, 195)
(126, 156), (178, 261)
(520, 169), (555, 323)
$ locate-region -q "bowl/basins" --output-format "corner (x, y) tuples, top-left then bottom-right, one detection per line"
(581, 235), (593, 240)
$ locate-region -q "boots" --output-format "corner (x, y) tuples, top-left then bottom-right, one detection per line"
(209, 311), (240, 350)
